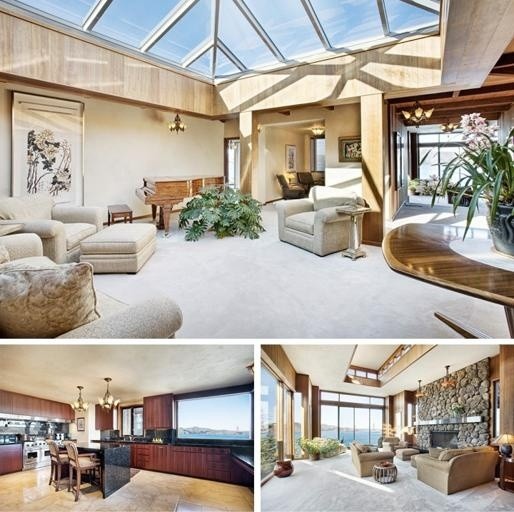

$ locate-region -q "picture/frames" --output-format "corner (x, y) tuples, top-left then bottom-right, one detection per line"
(284, 145), (297, 173)
(75, 417), (86, 432)
(337, 134), (361, 164)
(7, 89), (84, 210)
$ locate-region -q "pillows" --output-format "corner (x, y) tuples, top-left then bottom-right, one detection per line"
(439, 448), (474, 463)
(428, 448), (450, 461)
(0, 263), (101, 339)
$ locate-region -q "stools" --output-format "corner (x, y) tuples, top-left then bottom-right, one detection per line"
(104, 203), (134, 228)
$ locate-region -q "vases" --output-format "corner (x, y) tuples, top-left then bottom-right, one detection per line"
(487, 200), (514, 257)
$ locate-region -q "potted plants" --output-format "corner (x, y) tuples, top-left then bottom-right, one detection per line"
(322, 439), (340, 458)
(298, 436), (330, 461)
(178, 183), (267, 243)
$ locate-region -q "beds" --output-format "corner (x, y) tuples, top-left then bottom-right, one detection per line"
(381, 200), (514, 339)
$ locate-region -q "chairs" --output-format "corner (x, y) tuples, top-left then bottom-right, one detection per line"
(45, 440), (68, 492)
(63, 440), (106, 503)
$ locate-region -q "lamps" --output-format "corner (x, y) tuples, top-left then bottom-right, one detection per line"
(414, 379), (426, 397)
(494, 434), (514, 458)
(96, 378), (120, 413)
(399, 99), (435, 129)
(440, 117), (459, 138)
(165, 113), (187, 135)
(70, 385), (90, 413)
(304, 124), (325, 137)
(438, 366), (458, 389)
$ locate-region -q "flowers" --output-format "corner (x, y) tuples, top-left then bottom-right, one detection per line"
(429, 111), (514, 242)
(406, 174), (452, 201)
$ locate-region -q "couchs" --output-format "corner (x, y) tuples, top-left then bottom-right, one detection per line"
(396, 448), (420, 461)
(297, 170), (324, 194)
(0, 233), (182, 339)
(410, 445), (497, 496)
(72, 222), (161, 275)
(272, 186), (365, 258)
(0, 195), (101, 266)
(351, 440), (396, 478)
(273, 174), (307, 199)
